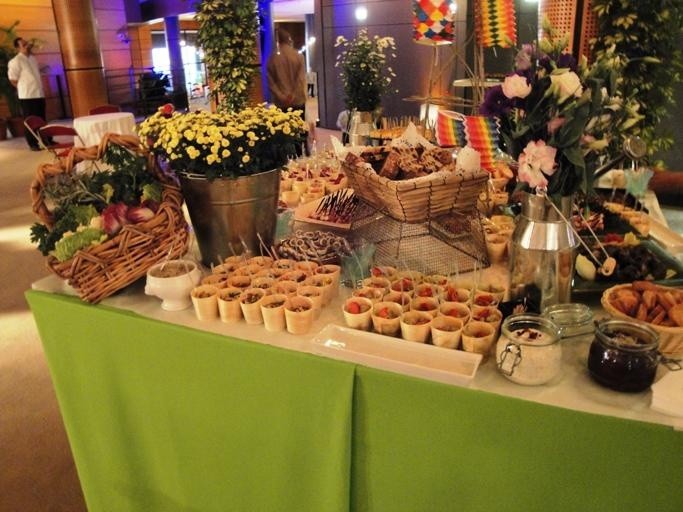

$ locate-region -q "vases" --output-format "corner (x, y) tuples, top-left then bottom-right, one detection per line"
(503, 194), (578, 309)
(345, 107), (377, 147)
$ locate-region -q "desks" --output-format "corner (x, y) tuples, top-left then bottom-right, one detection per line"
(71, 112), (135, 147)
(22, 222), (681, 510)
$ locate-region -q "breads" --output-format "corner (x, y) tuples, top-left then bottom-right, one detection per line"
(345, 142), (457, 177)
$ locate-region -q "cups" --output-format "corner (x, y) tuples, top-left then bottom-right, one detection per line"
(342, 263), (506, 366)
(190, 252), (341, 336)
(279, 143), (374, 208)
(471, 215), (517, 266)
(605, 201), (651, 240)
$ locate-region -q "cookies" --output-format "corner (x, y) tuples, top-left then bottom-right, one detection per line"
(613, 282), (682, 329)
(278, 229), (349, 259)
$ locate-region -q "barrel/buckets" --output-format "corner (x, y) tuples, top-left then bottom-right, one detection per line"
(513, 192), (572, 304)
(178, 168), (283, 270)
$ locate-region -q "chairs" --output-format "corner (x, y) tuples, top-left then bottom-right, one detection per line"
(88, 105), (121, 116)
(21, 113), (73, 164)
(35, 121), (101, 174)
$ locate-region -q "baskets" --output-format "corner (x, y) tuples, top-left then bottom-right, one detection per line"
(345, 199), (492, 278)
(337, 140), (489, 224)
(28, 133), (190, 306)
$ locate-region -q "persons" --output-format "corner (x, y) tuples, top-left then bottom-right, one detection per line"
(8, 37), (60, 151)
(266, 29), (307, 123)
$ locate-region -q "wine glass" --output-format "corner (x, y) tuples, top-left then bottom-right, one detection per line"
(143, 259), (202, 312)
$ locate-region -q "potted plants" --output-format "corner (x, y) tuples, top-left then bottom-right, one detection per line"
(4, 37), (51, 140)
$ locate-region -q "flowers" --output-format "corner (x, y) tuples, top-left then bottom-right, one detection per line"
(471, 10), (662, 226)
(18, 140), (165, 264)
(135, 99), (311, 188)
(331, 24), (404, 111)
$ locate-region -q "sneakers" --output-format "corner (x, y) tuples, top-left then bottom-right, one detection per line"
(30, 140), (58, 152)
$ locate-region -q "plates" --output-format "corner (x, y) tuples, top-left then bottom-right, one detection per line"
(295, 186), (382, 229)
(427, 219), (472, 240)
